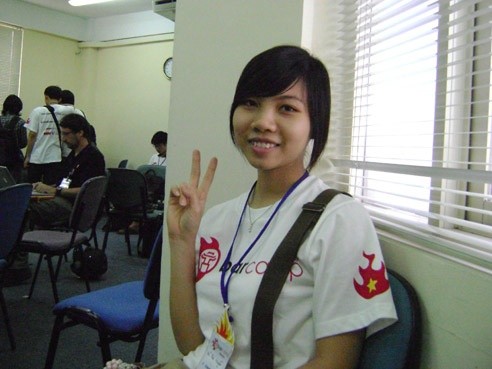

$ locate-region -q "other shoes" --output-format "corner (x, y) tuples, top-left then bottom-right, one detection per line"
(119, 225), (139, 235)
(3, 267), (33, 285)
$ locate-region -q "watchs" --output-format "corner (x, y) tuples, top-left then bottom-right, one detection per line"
(56, 187), (61, 195)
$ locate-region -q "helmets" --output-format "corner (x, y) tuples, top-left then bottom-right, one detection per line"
(71, 246), (108, 279)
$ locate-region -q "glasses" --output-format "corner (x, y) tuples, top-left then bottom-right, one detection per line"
(61, 131), (74, 136)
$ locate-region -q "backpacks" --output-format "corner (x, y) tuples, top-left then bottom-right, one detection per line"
(0, 115), (21, 163)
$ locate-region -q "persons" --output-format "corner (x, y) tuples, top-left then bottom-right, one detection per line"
(0, 86), (106, 287)
(118, 131), (168, 234)
(101, 45), (398, 369)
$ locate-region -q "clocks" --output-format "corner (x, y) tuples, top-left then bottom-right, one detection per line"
(163, 57), (172, 80)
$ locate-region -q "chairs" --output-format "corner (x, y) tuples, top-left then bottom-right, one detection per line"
(358, 267), (421, 369)
(0, 165), (165, 369)
